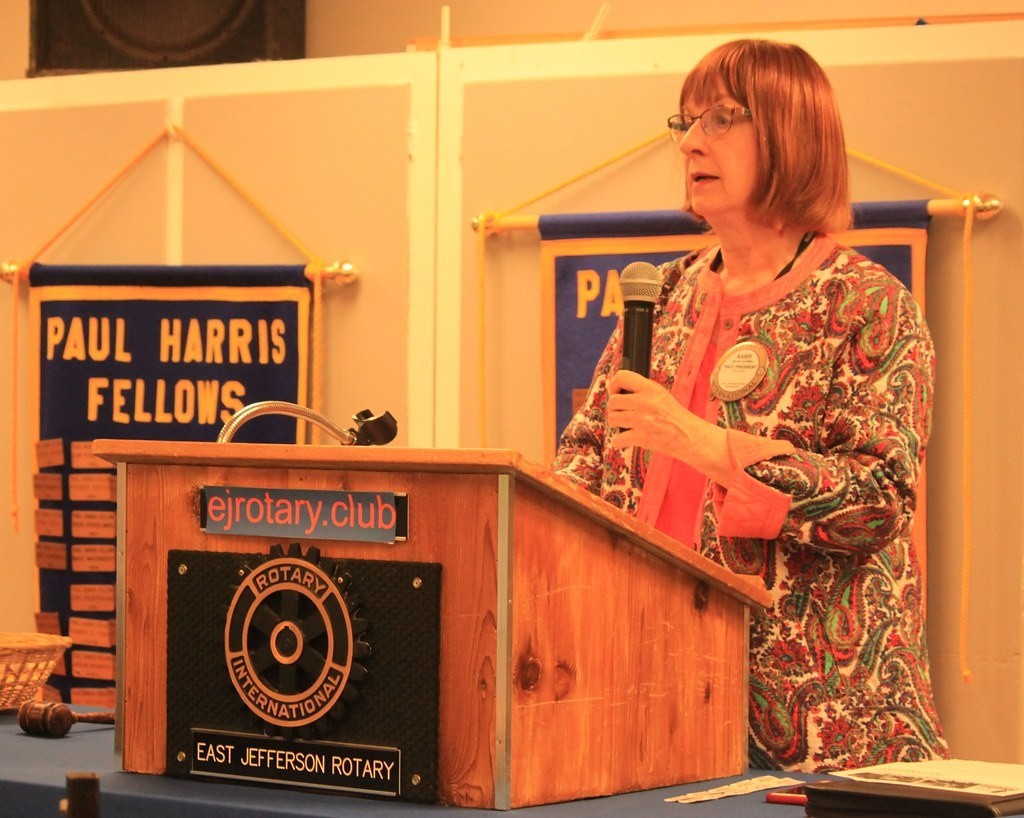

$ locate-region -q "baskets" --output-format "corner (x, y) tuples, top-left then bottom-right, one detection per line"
(0, 632), (73, 715)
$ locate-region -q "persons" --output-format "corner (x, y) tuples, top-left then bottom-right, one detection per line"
(551, 38), (951, 775)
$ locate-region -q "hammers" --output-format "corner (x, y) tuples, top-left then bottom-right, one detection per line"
(17, 698), (116, 740)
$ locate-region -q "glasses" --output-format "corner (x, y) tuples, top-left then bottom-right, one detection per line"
(667, 103), (753, 142)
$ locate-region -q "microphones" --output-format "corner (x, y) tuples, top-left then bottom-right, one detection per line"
(619, 260), (663, 434)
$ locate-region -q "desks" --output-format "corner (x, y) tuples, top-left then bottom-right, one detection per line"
(0, 705), (852, 818)
